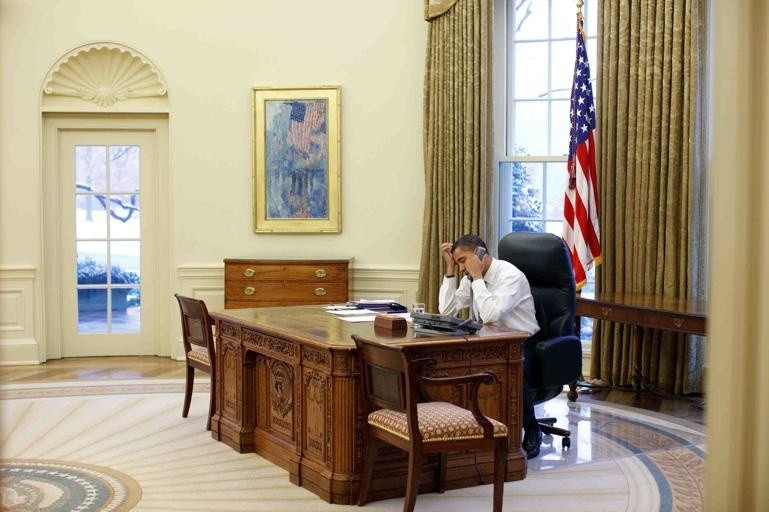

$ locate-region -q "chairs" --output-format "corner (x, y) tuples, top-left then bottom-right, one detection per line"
(497, 233), (583, 450)
(174, 294), (215, 431)
(352, 334), (508, 512)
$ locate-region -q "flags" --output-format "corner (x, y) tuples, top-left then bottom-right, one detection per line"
(287, 101), (325, 149)
(563, 13), (604, 293)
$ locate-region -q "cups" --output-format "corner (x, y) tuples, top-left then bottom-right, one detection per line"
(411, 302), (425, 328)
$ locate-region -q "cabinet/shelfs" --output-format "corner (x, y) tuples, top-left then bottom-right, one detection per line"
(223, 256), (355, 308)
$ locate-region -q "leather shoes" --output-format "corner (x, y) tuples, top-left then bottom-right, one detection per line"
(523, 427), (542, 459)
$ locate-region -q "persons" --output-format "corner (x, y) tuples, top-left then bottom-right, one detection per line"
(438, 234), (542, 460)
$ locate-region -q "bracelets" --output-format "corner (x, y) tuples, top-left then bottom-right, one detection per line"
(446, 273), (455, 278)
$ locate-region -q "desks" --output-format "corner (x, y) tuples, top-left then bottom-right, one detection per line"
(206, 301), (531, 505)
(576, 293), (708, 402)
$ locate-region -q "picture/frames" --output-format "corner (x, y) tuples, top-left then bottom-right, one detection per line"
(252, 85), (342, 236)
